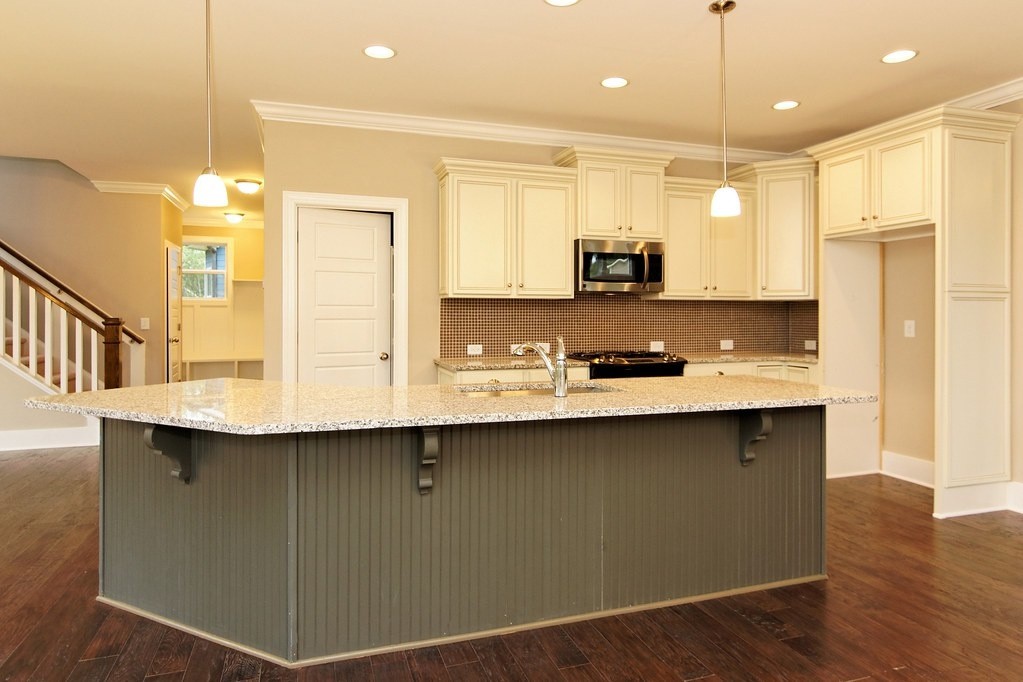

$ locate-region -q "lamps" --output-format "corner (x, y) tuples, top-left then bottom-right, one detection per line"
(706, 0), (742, 217)
(223, 213), (245, 224)
(192, 0), (228, 207)
(234, 179), (262, 194)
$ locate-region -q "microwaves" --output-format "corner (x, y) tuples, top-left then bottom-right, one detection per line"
(574, 239), (665, 295)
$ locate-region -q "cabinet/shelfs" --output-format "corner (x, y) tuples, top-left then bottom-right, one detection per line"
(641, 175), (757, 301)
(550, 145), (676, 245)
(432, 156), (577, 300)
(817, 104), (1023, 243)
(722, 157), (820, 301)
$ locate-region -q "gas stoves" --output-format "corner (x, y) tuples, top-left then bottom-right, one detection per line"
(567, 350), (688, 380)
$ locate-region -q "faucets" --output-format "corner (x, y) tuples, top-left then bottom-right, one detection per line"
(513, 336), (569, 398)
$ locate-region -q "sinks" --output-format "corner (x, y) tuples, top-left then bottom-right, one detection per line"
(452, 383), (628, 399)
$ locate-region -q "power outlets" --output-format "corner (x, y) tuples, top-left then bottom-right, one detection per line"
(535, 343), (550, 353)
(903, 320), (916, 337)
(719, 340), (734, 350)
(649, 341), (664, 352)
(466, 345), (483, 355)
(510, 344), (523, 355)
(805, 340), (817, 350)
